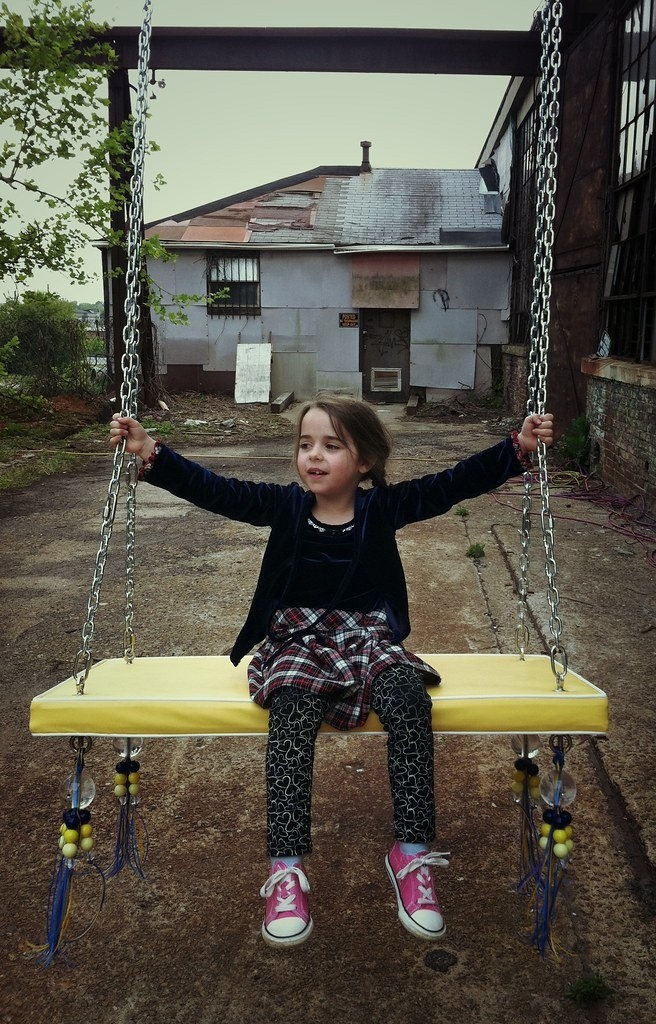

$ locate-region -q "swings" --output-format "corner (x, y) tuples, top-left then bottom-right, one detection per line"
(28, 0), (607, 737)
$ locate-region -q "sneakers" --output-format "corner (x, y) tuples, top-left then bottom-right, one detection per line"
(260, 860), (314, 949)
(385, 841), (451, 941)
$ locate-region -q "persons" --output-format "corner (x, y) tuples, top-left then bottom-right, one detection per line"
(109, 397), (554, 948)
